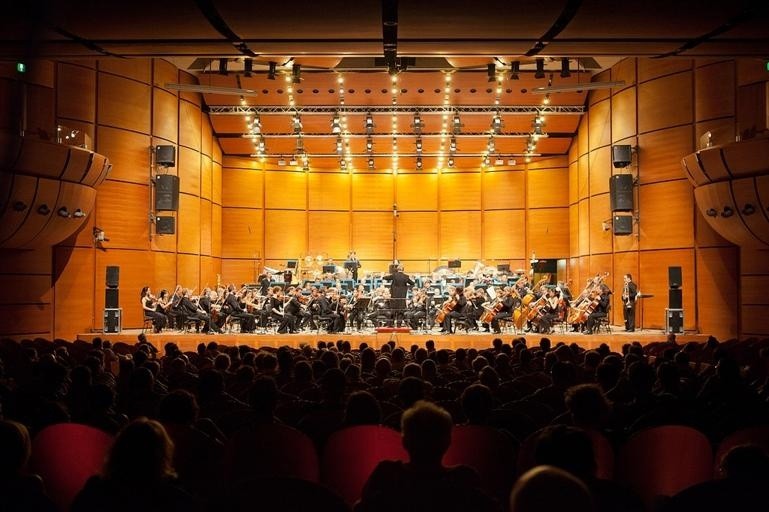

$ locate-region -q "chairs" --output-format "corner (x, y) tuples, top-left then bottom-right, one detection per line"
(440, 424), (501, 471)
(517, 423), (612, 484)
(220, 422), (321, 488)
(37, 423), (113, 489)
(324, 426), (413, 500)
(713, 425), (767, 481)
(140, 303), (615, 333)
(619, 422), (713, 498)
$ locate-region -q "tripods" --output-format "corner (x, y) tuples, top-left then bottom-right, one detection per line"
(635, 297), (650, 332)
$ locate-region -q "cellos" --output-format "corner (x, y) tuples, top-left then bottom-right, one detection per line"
(435, 271), (610, 329)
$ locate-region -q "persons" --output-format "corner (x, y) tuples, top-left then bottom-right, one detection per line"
(140, 275), (614, 336)
(1, 328), (769, 512)
(621, 273), (638, 333)
(381, 264), (414, 328)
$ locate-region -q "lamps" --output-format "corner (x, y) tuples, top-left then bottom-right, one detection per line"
(290, 63), (302, 84)
(267, 60), (277, 81)
(243, 58), (255, 78)
(217, 57), (230, 77)
(247, 111), (546, 171)
(533, 58), (546, 80)
(707, 204), (756, 217)
(386, 59), (408, 77)
(559, 58), (573, 78)
(14, 202), (85, 218)
(485, 62), (497, 83)
(509, 60), (522, 82)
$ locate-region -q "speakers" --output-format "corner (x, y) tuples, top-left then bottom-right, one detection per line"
(612, 145), (632, 168)
(613, 216), (632, 236)
(106, 288), (119, 308)
(106, 266), (119, 288)
(668, 289), (682, 309)
(668, 266), (682, 289)
(156, 145), (175, 167)
(664, 308), (683, 335)
(609, 174), (633, 212)
(102, 308), (122, 334)
(156, 175), (179, 211)
(156, 216), (175, 234)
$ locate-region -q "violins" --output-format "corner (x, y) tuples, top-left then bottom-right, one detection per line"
(297, 295), (310, 304)
(278, 296), (291, 302)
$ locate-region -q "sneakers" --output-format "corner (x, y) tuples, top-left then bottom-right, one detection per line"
(623, 329), (634, 332)
(156, 324), (361, 334)
(410, 321), (593, 335)
